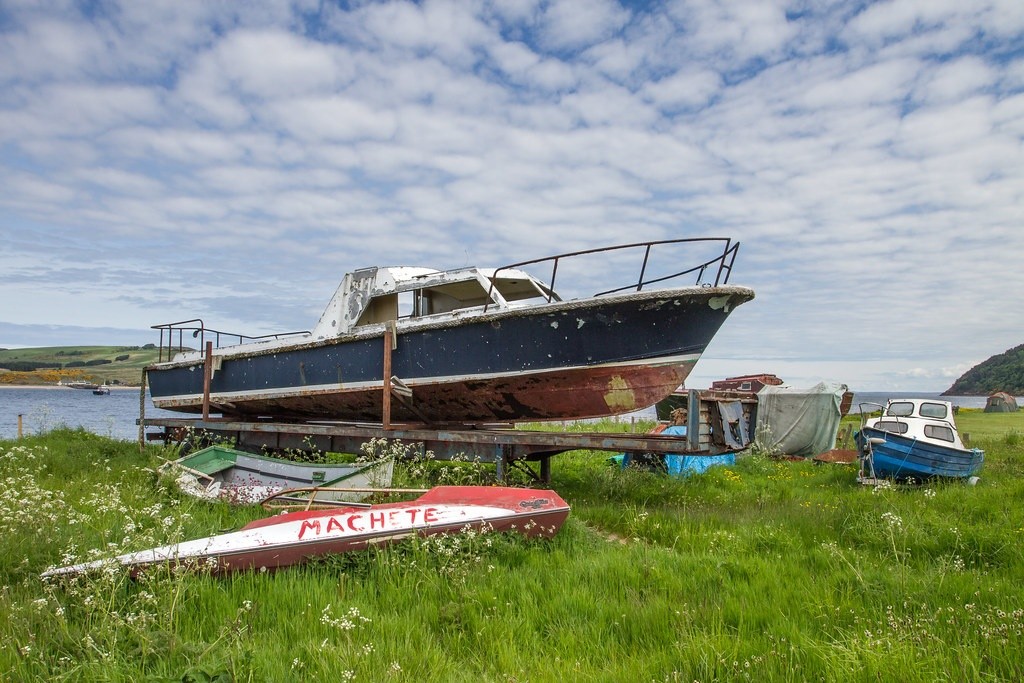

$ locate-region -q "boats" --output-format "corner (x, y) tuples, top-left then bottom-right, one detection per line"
(653, 373), (855, 460)
(67, 381), (111, 396)
(159, 446), (399, 514)
(144, 235), (755, 426)
(855, 398), (985, 486)
(38, 485), (570, 592)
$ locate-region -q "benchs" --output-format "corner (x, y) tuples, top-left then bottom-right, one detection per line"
(191, 457), (235, 479)
